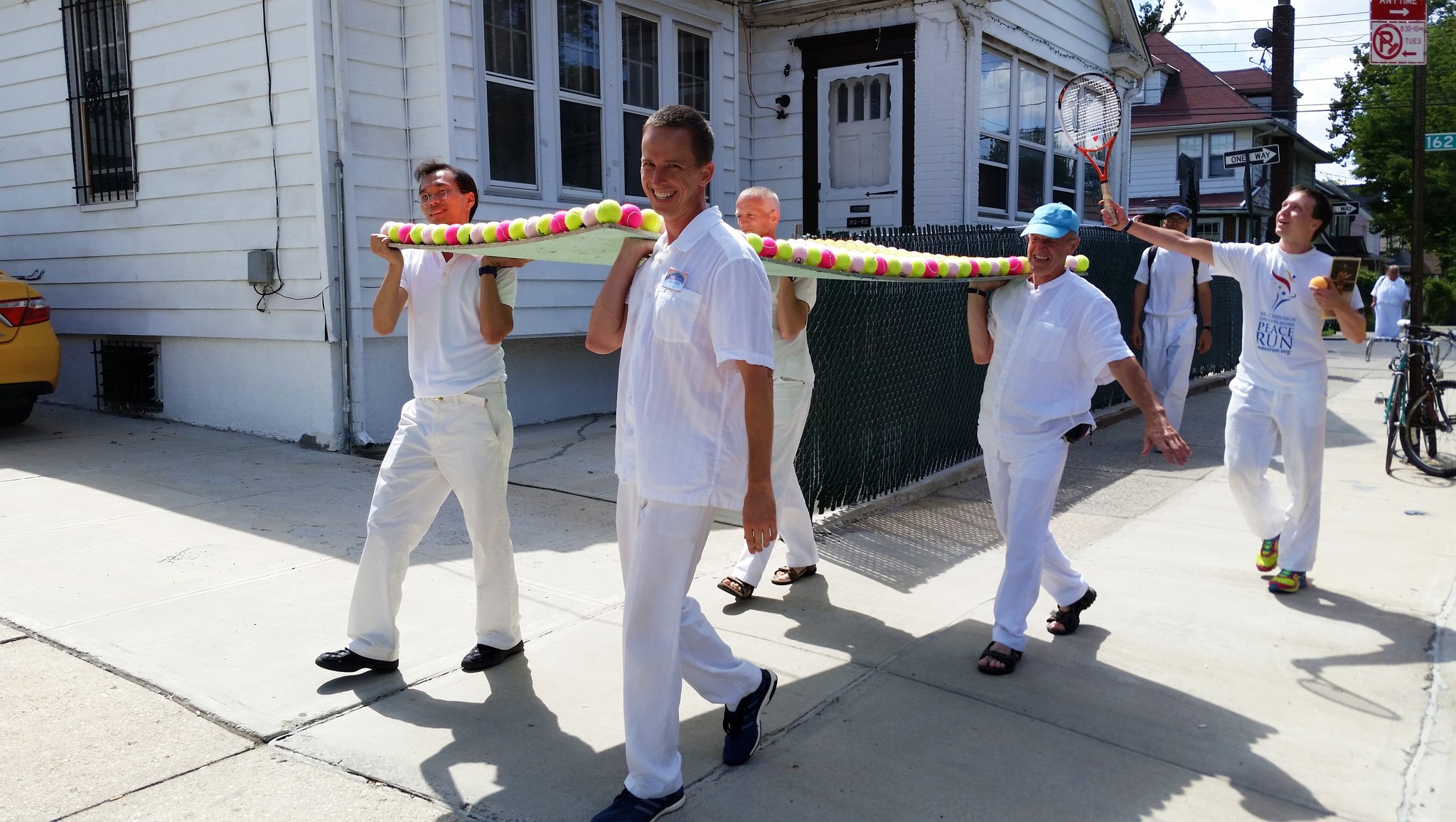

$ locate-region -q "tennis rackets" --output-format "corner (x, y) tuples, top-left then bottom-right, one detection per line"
(1056, 70), (1124, 226)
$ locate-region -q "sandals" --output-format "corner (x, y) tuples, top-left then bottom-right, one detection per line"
(717, 576), (754, 599)
(772, 563), (817, 585)
(978, 641), (1023, 674)
(1046, 587), (1096, 634)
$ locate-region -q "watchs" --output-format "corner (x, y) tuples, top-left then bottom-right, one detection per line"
(479, 265), (498, 278)
(786, 276), (797, 283)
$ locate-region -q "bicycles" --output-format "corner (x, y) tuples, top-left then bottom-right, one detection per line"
(1365, 324), (1456, 478)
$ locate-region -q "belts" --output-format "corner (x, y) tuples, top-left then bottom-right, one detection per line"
(427, 383), (506, 408)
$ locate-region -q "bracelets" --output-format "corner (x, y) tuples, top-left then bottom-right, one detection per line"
(966, 286), (988, 298)
(1121, 218), (1133, 233)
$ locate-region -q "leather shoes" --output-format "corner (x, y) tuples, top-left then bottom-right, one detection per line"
(461, 639), (523, 669)
(315, 648), (399, 673)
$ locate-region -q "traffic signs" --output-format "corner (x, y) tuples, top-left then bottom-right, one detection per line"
(1330, 201), (1360, 216)
(1223, 144), (1280, 170)
(1369, 0), (1428, 66)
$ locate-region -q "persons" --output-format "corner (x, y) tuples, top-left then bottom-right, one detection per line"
(315, 163), (525, 673)
(965, 203), (1191, 674)
(1337, 265), (1348, 295)
(1371, 264), (1411, 339)
(1097, 184), (1367, 593)
(1132, 206), (1216, 454)
(582, 106), (777, 822)
(717, 186), (816, 599)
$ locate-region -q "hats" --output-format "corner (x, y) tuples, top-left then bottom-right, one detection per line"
(1165, 205), (1189, 219)
(1020, 202), (1079, 239)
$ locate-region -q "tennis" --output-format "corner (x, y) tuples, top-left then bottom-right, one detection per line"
(379, 197), (1090, 279)
(1309, 276), (1329, 289)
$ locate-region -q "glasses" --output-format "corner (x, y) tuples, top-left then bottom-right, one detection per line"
(1024, 234), (1074, 245)
(1165, 218), (1186, 224)
(414, 188), (470, 206)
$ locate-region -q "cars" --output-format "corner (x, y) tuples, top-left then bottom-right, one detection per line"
(0, 272), (61, 430)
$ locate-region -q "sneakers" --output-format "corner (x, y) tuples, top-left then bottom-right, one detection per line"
(1268, 569), (1307, 592)
(722, 668), (778, 765)
(591, 784), (685, 822)
(1256, 533), (1282, 571)
